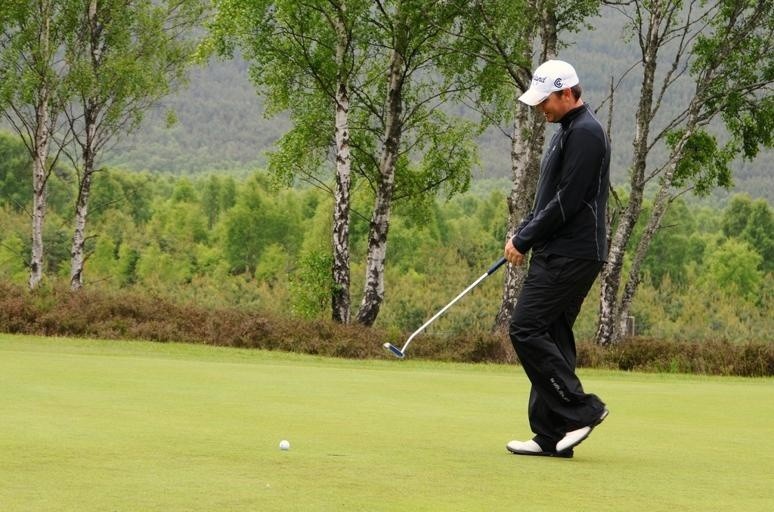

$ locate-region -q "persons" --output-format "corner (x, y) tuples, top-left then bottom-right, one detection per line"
(506, 59), (609, 456)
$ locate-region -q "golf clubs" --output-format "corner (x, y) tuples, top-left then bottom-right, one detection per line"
(383, 258), (507, 359)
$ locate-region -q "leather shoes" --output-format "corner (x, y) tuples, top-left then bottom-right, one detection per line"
(555, 409), (609, 453)
(507, 435), (573, 458)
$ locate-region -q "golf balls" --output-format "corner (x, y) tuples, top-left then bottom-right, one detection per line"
(279, 440), (290, 451)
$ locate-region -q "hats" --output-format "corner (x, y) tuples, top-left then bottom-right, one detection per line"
(518, 60), (579, 107)
